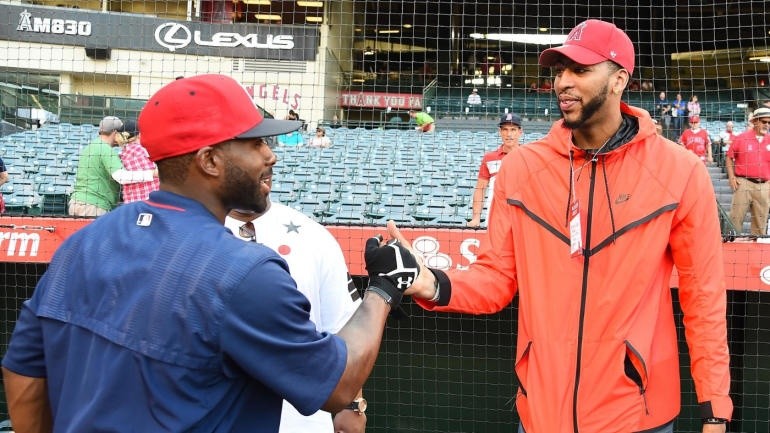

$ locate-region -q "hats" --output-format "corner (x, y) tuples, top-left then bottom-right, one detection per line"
(99, 116), (123, 132)
(140, 75), (303, 162)
(545, 21), (635, 76)
(120, 120), (139, 139)
(690, 117), (699, 123)
(749, 108), (770, 123)
(498, 113), (521, 130)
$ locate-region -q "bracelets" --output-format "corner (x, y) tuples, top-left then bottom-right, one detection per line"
(703, 419), (724, 423)
(428, 283), (440, 302)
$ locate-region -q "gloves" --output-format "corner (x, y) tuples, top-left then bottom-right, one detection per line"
(365, 234), (420, 308)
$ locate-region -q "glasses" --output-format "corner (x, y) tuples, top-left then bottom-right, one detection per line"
(762, 121), (770, 124)
(240, 221), (256, 244)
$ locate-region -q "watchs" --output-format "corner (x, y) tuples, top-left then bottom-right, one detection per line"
(346, 399), (367, 414)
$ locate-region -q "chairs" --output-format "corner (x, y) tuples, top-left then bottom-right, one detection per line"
(0, 121), (547, 227)
(426, 95), (745, 121)
(699, 122), (747, 171)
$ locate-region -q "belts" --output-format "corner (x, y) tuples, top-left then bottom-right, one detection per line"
(739, 175), (765, 183)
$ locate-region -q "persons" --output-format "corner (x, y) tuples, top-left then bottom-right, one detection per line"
(680, 116), (713, 163)
(119, 121), (160, 203)
(0, 158), (9, 211)
(387, 19), (734, 433)
(655, 92), (701, 142)
(725, 106), (770, 236)
(222, 197), (367, 432)
(330, 115), (342, 129)
(468, 89), (481, 104)
(744, 99), (770, 131)
(468, 114), (522, 226)
(408, 110), (435, 132)
(625, 79), (653, 90)
(530, 79), (550, 92)
(312, 126), (331, 145)
(0, 74), (420, 433)
(715, 121), (735, 151)
(278, 110), (303, 146)
(68, 116), (159, 216)
